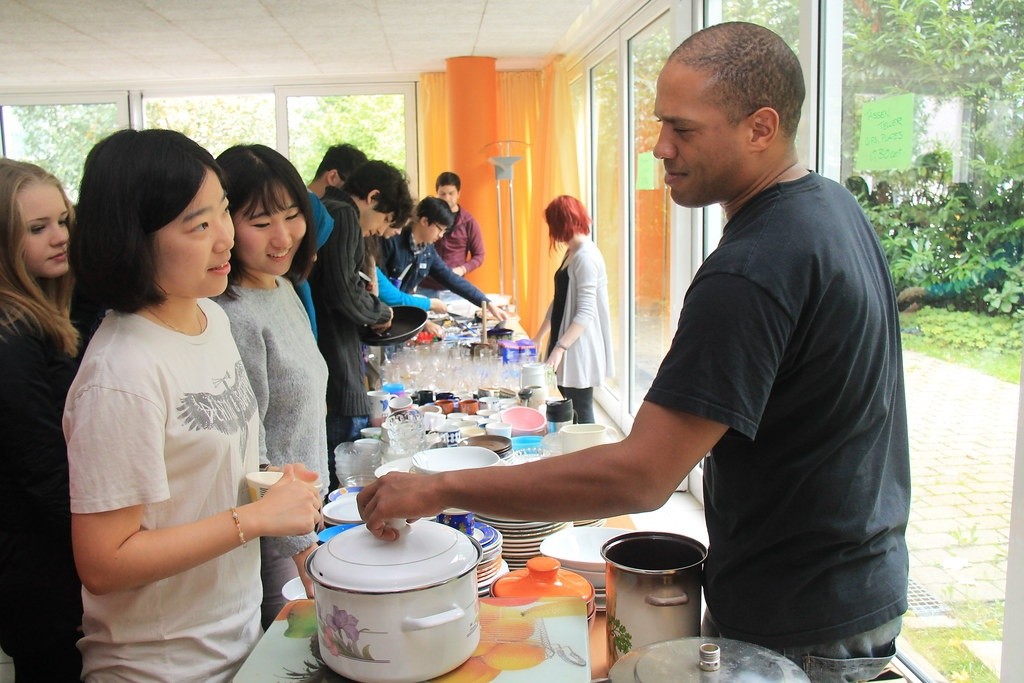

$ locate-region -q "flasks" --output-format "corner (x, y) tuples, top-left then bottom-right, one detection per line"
(546, 399), (578, 432)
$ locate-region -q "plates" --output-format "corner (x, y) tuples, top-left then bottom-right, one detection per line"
(594, 589), (606, 612)
(317, 486), (366, 542)
(474, 512), (606, 571)
(457, 435), (513, 462)
(374, 457), (412, 478)
(471, 521), (509, 597)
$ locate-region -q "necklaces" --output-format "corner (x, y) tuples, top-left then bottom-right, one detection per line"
(145, 306), (203, 336)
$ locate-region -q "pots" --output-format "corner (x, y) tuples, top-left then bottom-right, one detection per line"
(359, 306), (427, 345)
(600, 532), (708, 676)
(491, 557), (596, 632)
(304, 518), (483, 683)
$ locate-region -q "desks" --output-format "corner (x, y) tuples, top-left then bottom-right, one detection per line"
(229, 321), (638, 683)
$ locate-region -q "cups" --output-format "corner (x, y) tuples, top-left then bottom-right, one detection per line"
(381, 340), (545, 388)
(557, 424), (606, 454)
(436, 508), (474, 536)
(366, 382), (547, 447)
(245, 472), (324, 503)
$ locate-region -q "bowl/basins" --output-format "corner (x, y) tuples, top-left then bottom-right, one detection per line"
(501, 407), (545, 436)
(539, 527), (636, 587)
(511, 436), (544, 453)
(334, 442), (381, 486)
(412, 446), (501, 474)
(282, 577), (315, 605)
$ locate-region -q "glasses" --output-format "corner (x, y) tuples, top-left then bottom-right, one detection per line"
(434, 223), (449, 235)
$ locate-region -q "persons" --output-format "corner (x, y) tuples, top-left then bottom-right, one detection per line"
(532, 195), (614, 424)
(357, 22), (912, 683)
(0, 131), (508, 683)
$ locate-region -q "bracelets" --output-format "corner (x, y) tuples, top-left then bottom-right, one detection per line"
(231, 507), (248, 544)
(556, 341), (567, 350)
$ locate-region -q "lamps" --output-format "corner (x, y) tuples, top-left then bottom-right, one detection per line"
(479, 140), (532, 297)
